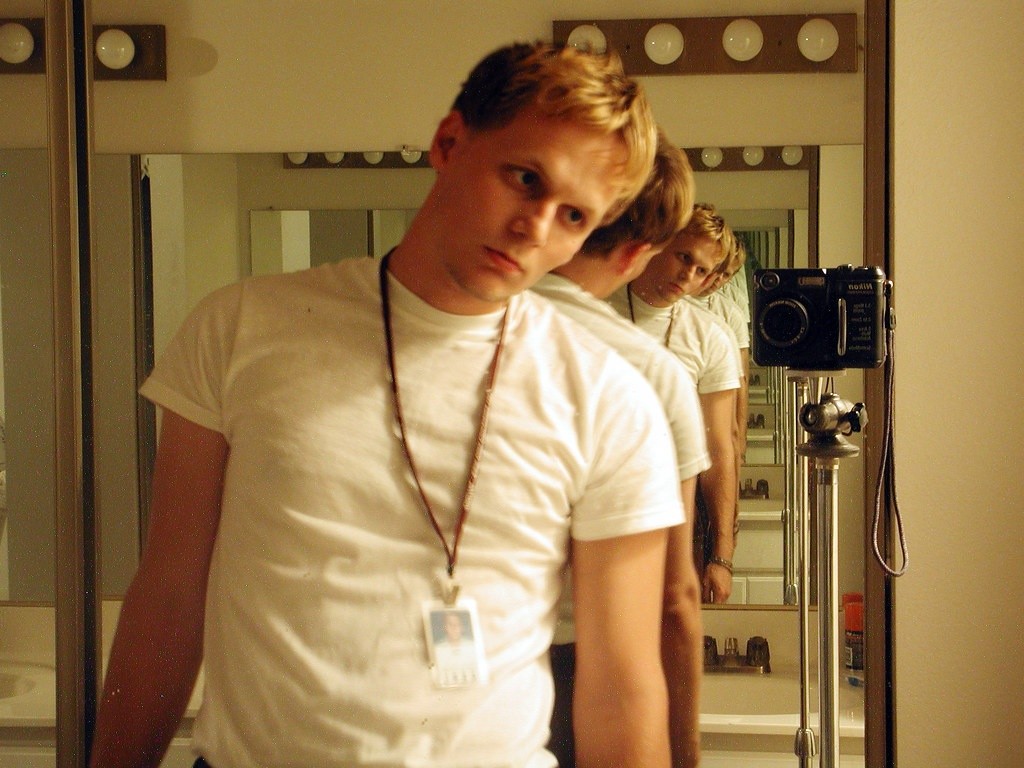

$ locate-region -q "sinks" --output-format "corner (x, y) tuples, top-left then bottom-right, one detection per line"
(739, 499), (784, 512)
(748, 429), (774, 436)
(749, 385), (767, 390)
(697, 671), (858, 715)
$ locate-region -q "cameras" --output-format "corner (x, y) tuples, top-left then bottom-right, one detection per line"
(753, 264), (896, 373)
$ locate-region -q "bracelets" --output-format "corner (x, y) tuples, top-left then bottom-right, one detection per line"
(710, 554), (734, 573)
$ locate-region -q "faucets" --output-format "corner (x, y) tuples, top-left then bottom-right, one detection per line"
(749, 375), (753, 385)
(748, 414), (755, 427)
(745, 478), (753, 494)
(724, 638), (738, 667)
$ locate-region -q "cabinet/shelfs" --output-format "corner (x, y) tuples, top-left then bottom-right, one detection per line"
(676, 352), (833, 750)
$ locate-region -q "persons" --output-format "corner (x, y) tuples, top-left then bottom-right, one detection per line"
(518, 129), (753, 766)
(72, 34), (677, 766)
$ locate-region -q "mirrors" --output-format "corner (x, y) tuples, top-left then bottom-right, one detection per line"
(66, 18), (895, 768)
